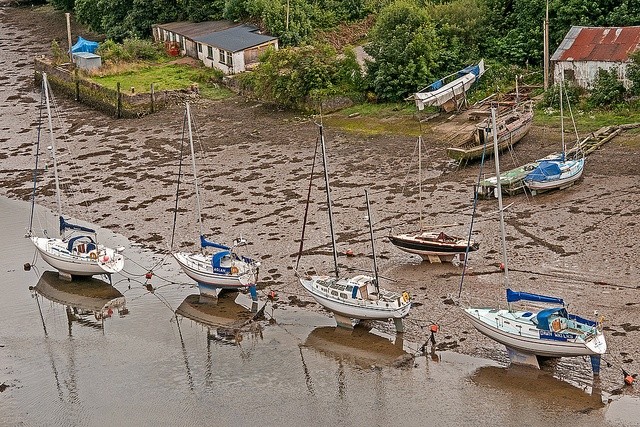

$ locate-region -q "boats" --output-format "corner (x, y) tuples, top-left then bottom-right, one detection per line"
(447, 100), (534, 160)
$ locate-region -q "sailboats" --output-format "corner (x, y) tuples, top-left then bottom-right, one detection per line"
(22, 72), (126, 283)
(169, 99), (262, 307)
(387, 134), (480, 263)
(462, 362), (604, 425)
(169, 292), (269, 400)
(299, 327), (417, 407)
(30, 271), (128, 404)
(523, 61), (586, 197)
(455, 107), (608, 377)
(296, 100), (412, 335)
